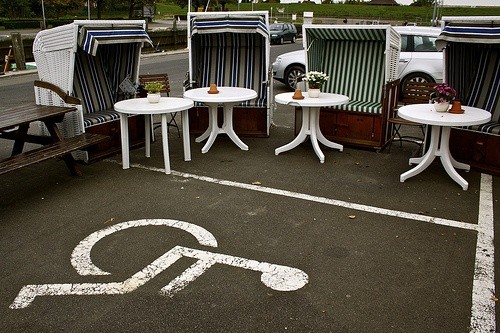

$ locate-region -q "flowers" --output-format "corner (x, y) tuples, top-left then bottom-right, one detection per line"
(301, 68), (331, 90)
(430, 82), (458, 103)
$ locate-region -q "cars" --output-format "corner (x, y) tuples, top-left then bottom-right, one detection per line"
(270, 23), (297, 45)
(273, 27), (444, 96)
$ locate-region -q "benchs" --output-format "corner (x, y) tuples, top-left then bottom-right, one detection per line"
(74, 47), (156, 166)
(0, 128), (112, 177)
(184, 46), (269, 140)
(310, 41), (386, 150)
(442, 48), (500, 175)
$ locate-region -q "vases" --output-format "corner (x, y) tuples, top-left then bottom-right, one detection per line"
(308, 88), (321, 97)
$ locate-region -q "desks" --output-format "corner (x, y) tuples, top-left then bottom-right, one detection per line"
(114, 97), (194, 175)
(0, 103), (85, 178)
(183, 85), (258, 154)
(394, 103), (493, 191)
(274, 91), (350, 164)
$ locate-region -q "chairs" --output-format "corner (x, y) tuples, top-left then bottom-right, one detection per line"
(419, 37), (432, 51)
(382, 78), (427, 156)
(138, 73), (182, 137)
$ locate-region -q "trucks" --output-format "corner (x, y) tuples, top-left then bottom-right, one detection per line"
(129, 0), (154, 22)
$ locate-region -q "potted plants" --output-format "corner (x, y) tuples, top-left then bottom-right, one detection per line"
(143, 80), (165, 104)
